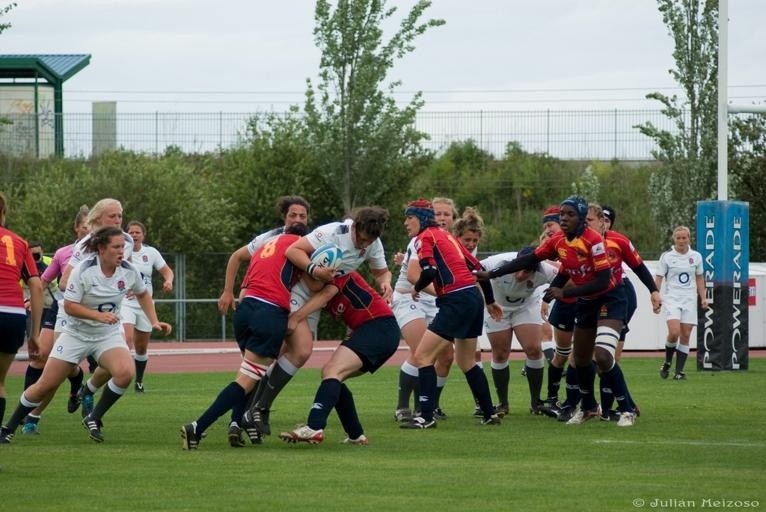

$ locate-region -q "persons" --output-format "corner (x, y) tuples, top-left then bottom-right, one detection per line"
(0, 197), (175, 444)
(654, 226), (708, 379)
(180, 195), (662, 451)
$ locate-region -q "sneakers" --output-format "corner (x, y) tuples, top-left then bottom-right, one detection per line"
(343, 434), (369, 445)
(530, 397), (639, 426)
(473, 402), (508, 425)
(392, 407), (446, 429)
(76, 388), (93, 419)
(20, 414), (38, 435)
(659, 361), (671, 379)
(278, 426), (325, 445)
(180, 421), (207, 451)
(68, 385), (82, 413)
(134, 382), (144, 393)
(228, 403), (271, 447)
(673, 371), (686, 380)
(82, 412), (103, 442)
(0, 424), (13, 444)
